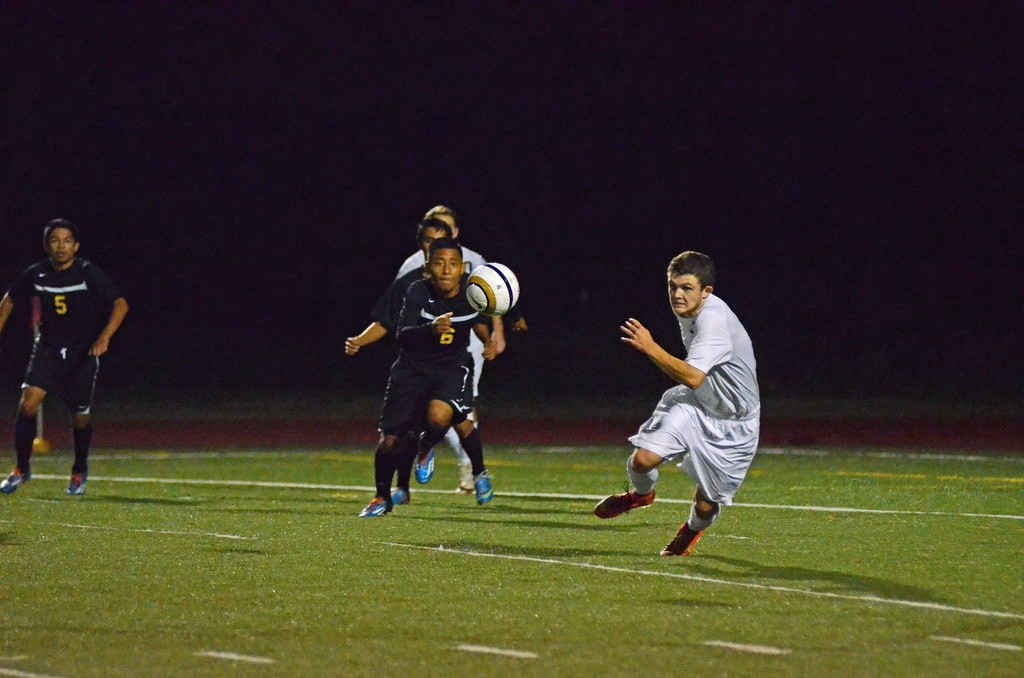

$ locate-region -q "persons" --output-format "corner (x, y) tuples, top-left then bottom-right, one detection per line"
(595, 251), (759, 559)
(0, 218), (128, 497)
(345, 206), (527, 518)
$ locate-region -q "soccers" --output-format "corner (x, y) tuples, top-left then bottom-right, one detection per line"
(462, 260), (522, 316)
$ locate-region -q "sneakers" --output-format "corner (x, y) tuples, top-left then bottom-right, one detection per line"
(473, 469), (494, 505)
(660, 521), (704, 557)
(0, 468), (32, 494)
(66, 466), (88, 496)
(459, 462), (474, 491)
(359, 497), (392, 517)
(415, 432), (434, 484)
(594, 481), (656, 519)
(390, 490), (412, 503)
